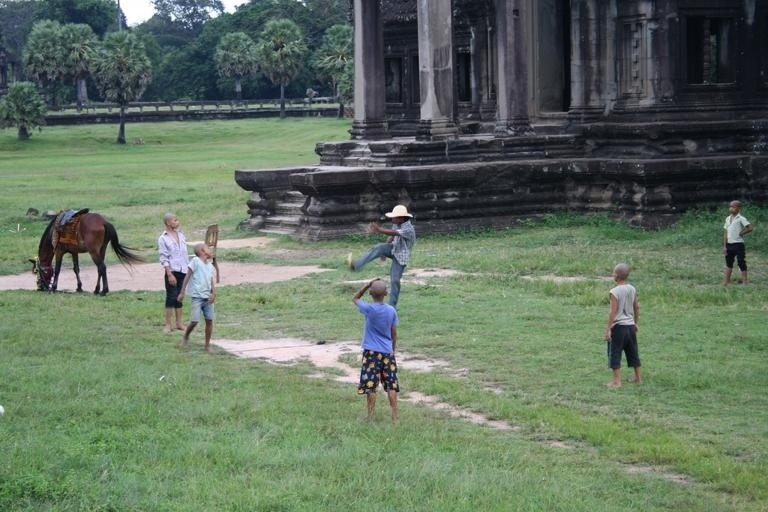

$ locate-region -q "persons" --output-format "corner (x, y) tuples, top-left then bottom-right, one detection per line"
(351, 277), (399, 424)
(604, 261), (643, 388)
(343, 205), (416, 308)
(722, 200), (753, 285)
(157, 212), (189, 333)
(176, 243), (217, 353)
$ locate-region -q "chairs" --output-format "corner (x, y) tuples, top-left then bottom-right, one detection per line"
(188, 224), (219, 282)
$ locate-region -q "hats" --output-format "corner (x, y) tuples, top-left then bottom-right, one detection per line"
(385, 205), (414, 218)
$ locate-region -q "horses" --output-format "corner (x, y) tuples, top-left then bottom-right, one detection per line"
(26, 210), (147, 297)
